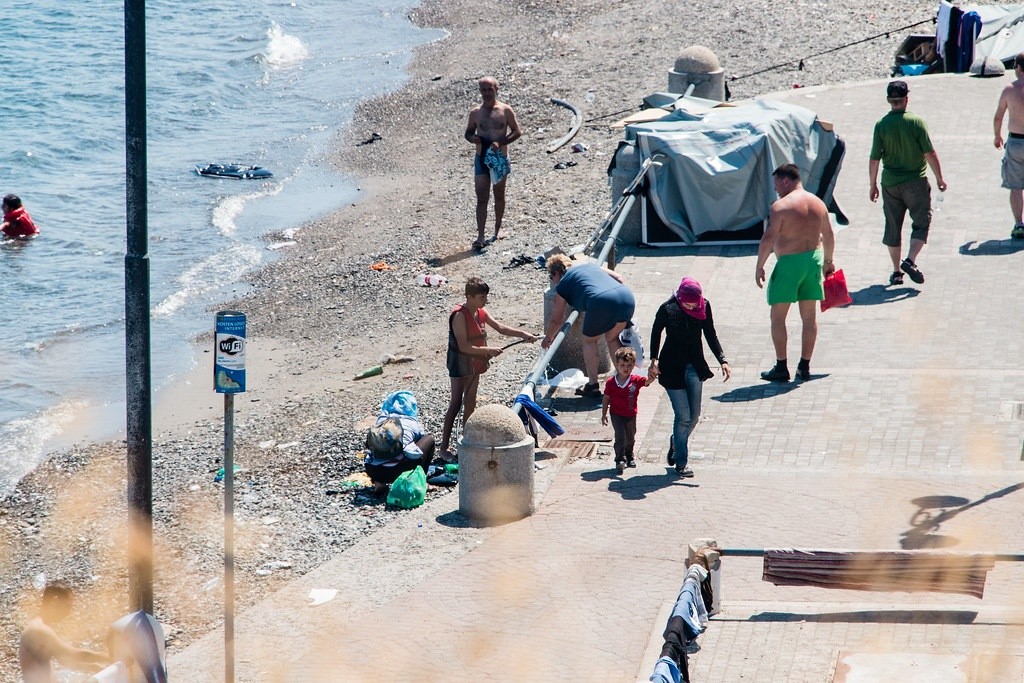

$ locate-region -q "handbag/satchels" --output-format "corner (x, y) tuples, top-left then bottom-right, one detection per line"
(619, 325), (645, 369)
(819, 269), (851, 313)
(484, 147), (511, 185)
(386, 465), (426, 508)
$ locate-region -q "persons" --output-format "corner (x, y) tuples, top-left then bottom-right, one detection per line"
(601, 346), (659, 471)
(869, 81), (948, 287)
(992, 53), (1024, 238)
(542, 254), (636, 394)
(438, 276), (536, 465)
(0, 194), (36, 239)
(650, 276), (731, 477)
(363, 391), (436, 496)
(464, 76), (522, 248)
(754, 164), (836, 380)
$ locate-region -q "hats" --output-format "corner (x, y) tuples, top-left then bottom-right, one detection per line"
(886, 81), (910, 98)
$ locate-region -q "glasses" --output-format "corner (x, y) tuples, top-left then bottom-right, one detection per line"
(549, 275), (553, 280)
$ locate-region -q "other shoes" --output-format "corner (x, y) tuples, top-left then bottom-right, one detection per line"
(626, 460), (636, 468)
(674, 466), (694, 477)
(795, 366), (809, 379)
(901, 259), (924, 284)
(890, 271), (905, 284)
(486, 235), (500, 245)
(666, 433), (676, 466)
(1011, 223), (1024, 238)
(760, 365), (790, 381)
(472, 237), (485, 247)
(616, 460), (627, 471)
(440, 449), (453, 462)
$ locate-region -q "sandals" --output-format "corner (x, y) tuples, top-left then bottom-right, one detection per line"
(574, 381), (602, 396)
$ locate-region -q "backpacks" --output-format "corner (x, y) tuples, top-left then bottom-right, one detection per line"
(365, 416), (405, 459)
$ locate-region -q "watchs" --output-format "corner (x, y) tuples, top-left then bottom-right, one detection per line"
(825, 260), (833, 263)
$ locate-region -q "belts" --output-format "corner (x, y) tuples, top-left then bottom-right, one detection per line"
(1008, 133), (1024, 139)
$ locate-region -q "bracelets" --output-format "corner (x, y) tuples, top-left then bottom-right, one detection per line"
(544, 335), (554, 345)
